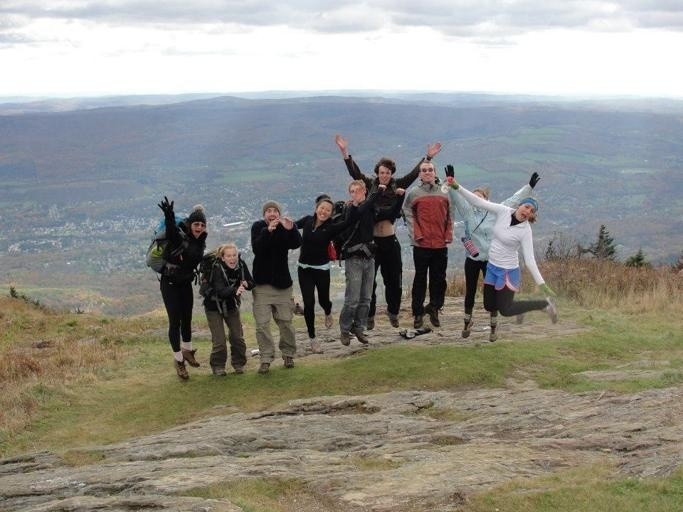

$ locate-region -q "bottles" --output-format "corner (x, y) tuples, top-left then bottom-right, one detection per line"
(441, 177), (455, 194)
(150, 244), (164, 256)
(462, 238), (479, 259)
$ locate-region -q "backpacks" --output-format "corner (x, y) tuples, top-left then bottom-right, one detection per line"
(199, 251), (217, 299)
(332, 200), (348, 261)
(146, 214), (188, 276)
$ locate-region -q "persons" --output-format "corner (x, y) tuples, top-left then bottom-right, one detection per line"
(446, 166), (541, 340)
(401, 162), (454, 328)
(445, 176), (558, 322)
(250, 200), (302, 374)
(292, 184), (367, 338)
(338, 179), (406, 344)
(336, 132), (442, 330)
(205, 243), (256, 377)
(154, 197), (207, 380)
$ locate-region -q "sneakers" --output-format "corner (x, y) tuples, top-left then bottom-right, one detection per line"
(488, 321), (499, 342)
(462, 317), (474, 338)
(425, 302), (440, 327)
(282, 353), (294, 368)
(350, 324), (368, 343)
(234, 364), (244, 374)
(413, 306), (426, 329)
(212, 366), (226, 376)
(182, 347), (200, 367)
(258, 362), (270, 373)
(367, 315), (375, 330)
(311, 337), (323, 354)
(340, 329), (350, 346)
(174, 357), (189, 379)
(325, 313), (332, 328)
(542, 296), (558, 324)
(388, 312), (399, 328)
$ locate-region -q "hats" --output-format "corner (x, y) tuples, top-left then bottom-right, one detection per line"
(262, 200), (281, 217)
(189, 210), (207, 224)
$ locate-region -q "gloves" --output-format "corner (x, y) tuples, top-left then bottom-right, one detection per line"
(529, 171), (541, 188)
(447, 180), (459, 190)
(158, 195), (175, 215)
(540, 283), (556, 298)
(445, 164), (454, 180)
